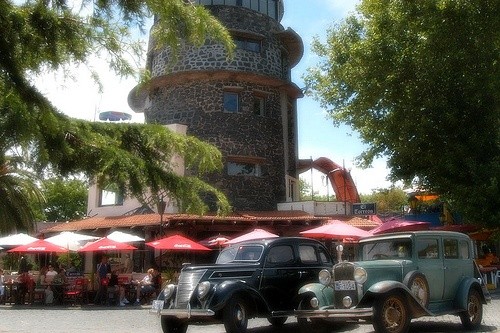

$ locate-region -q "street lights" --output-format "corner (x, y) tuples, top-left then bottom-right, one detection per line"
(408, 193), (419, 220)
(157, 195), (166, 271)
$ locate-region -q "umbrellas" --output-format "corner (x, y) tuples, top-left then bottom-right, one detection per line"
(370, 217), (430, 234)
(224, 229), (279, 244)
(44, 231), (101, 271)
(107, 231), (145, 243)
(7, 239), (69, 272)
(145, 235), (212, 267)
(346, 217), (381, 226)
(198, 233), (232, 246)
(0, 233), (39, 250)
(78, 236), (138, 255)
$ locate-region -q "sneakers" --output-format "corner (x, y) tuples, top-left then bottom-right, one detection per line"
(118, 298), (129, 306)
(134, 300), (141, 305)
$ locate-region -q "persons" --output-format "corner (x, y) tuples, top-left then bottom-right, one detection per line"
(25, 264), (36, 307)
(36, 264), (69, 306)
(95, 254), (129, 308)
(133, 268), (162, 306)
(0, 269), (8, 305)
(299, 220), (374, 262)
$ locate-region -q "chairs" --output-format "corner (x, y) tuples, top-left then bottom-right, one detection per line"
(66, 285), (82, 306)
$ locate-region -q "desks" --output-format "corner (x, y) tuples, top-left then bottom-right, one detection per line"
(119, 284), (137, 305)
(3, 283), (18, 305)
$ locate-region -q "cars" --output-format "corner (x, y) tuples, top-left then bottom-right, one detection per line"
(148, 237), (333, 333)
(274, 230), (492, 333)
